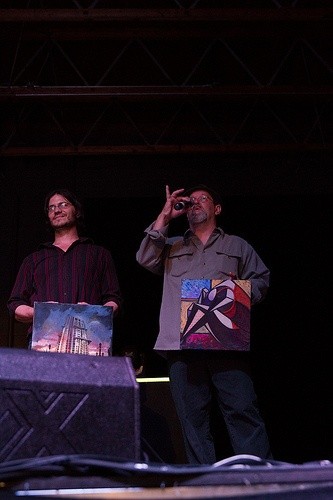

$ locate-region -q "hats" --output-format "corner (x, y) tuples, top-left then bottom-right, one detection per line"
(182, 184), (222, 205)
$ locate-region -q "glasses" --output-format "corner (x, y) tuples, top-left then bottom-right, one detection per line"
(47, 202), (73, 212)
(190, 195), (214, 202)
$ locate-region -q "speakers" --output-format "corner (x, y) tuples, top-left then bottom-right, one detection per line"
(0, 347), (142, 463)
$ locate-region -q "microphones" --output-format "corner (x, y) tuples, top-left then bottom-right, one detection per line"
(174, 199), (195, 210)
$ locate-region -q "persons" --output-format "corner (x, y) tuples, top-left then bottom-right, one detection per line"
(136, 185), (274, 465)
(7, 191), (123, 351)
